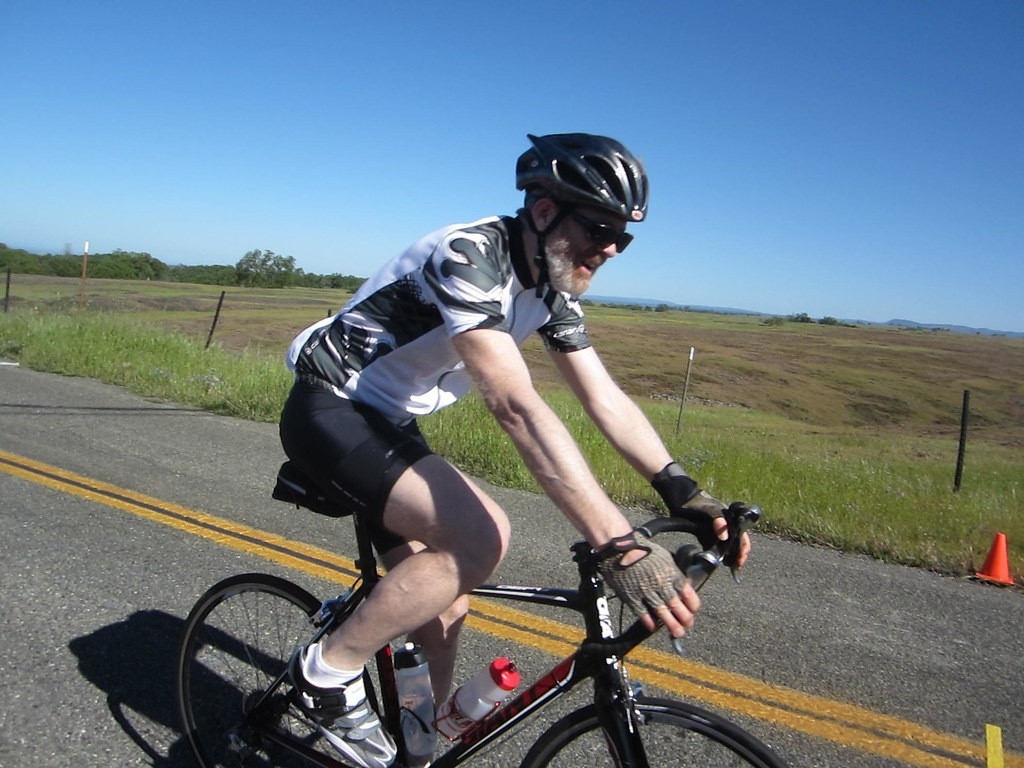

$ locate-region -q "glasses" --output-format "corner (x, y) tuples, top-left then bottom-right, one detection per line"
(568, 204), (638, 254)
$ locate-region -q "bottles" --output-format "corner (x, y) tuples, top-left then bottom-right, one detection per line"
(392, 638), (441, 759)
(436, 657), (520, 738)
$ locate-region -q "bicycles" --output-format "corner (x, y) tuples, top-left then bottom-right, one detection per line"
(172, 461), (790, 767)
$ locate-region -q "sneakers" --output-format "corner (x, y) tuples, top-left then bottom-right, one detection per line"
(287, 647), (398, 765)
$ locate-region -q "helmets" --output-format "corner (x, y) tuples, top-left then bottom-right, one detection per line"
(514, 133), (651, 217)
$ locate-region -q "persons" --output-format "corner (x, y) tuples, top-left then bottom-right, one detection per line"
(278, 131), (749, 768)
